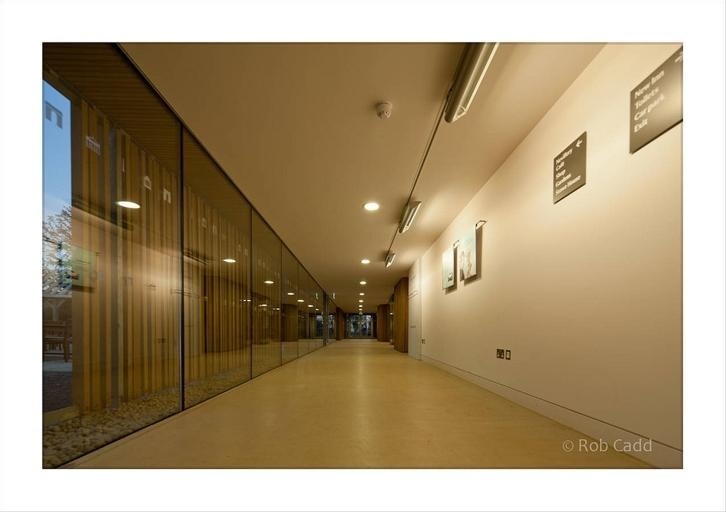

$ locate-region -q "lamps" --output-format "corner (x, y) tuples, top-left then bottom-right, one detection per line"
(398, 199), (424, 236)
(443, 42), (499, 125)
